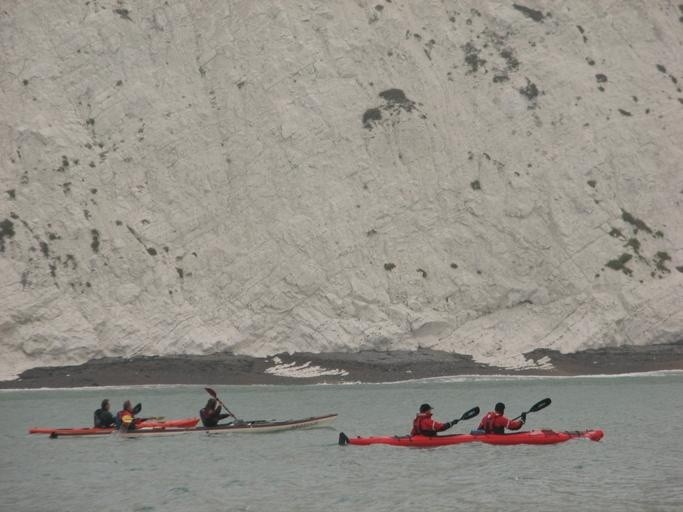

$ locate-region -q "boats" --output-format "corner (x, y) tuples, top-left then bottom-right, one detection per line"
(114, 413), (337, 438)
(28, 418), (200, 438)
(339, 428), (605, 447)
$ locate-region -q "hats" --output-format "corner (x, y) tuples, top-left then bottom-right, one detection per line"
(496, 402), (505, 408)
(420, 404), (434, 409)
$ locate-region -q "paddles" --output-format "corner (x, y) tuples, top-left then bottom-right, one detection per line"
(512, 398), (551, 421)
(147, 417), (164, 420)
(133, 403), (141, 414)
(453, 406), (480, 423)
(205, 388), (237, 420)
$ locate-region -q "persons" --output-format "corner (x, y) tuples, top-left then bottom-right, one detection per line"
(115, 400), (145, 430)
(92, 398), (117, 429)
(199, 397), (234, 427)
(409, 403), (458, 437)
(476, 402), (526, 435)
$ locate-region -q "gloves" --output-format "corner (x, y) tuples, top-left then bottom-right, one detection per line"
(521, 412), (526, 423)
(445, 420), (458, 429)
